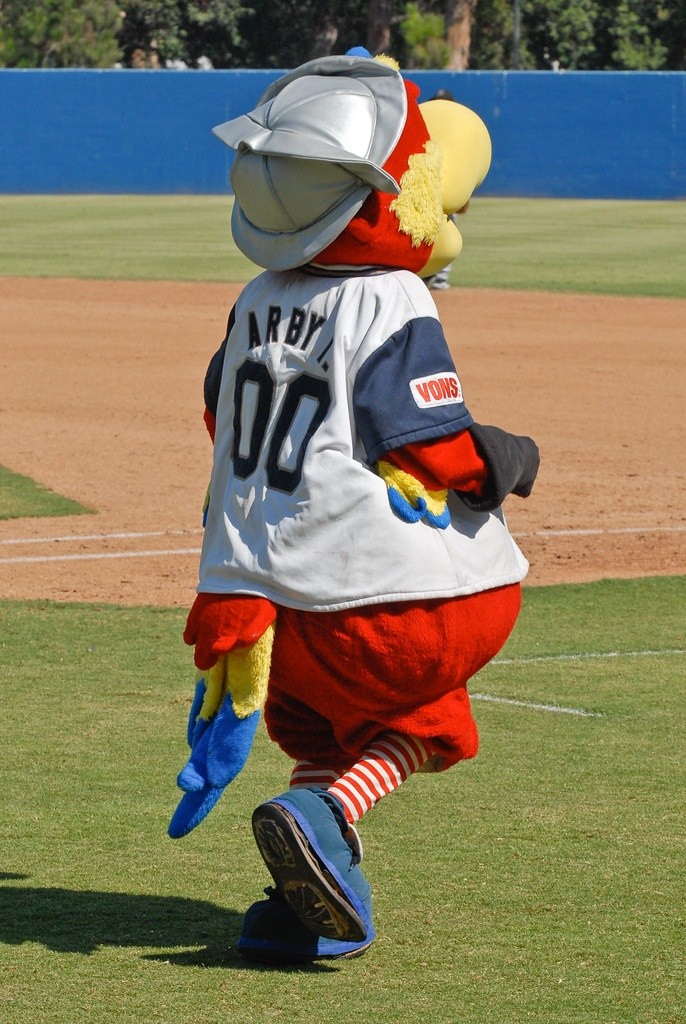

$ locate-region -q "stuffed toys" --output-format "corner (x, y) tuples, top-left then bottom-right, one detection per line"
(168, 47), (539, 958)
